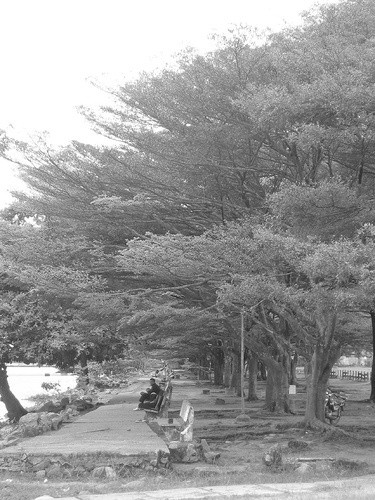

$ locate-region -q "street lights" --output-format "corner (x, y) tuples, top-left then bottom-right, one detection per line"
(236, 306), (249, 421)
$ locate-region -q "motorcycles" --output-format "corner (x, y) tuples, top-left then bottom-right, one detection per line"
(323, 391), (344, 426)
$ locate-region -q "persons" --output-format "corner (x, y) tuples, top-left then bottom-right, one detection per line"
(326, 396), (335, 412)
(134, 388), (157, 412)
(155, 367), (168, 377)
(139, 378), (162, 407)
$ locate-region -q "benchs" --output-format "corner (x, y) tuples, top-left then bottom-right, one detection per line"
(158, 400), (193, 444)
(145, 397), (169, 418)
